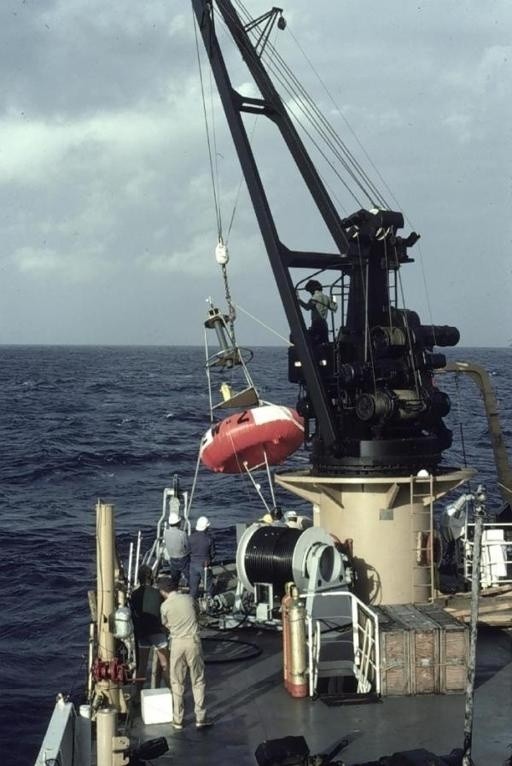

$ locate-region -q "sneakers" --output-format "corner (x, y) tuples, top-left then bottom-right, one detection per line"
(173, 721), (183, 729)
(196, 718), (215, 727)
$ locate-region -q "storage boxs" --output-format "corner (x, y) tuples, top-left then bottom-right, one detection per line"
(358, 602), (471, 697)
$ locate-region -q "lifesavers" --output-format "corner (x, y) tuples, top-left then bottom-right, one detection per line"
(427, 530), (442, 570)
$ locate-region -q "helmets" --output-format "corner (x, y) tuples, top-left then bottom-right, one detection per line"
(196, 517), (210, 531)
(169, 513), (181, 524)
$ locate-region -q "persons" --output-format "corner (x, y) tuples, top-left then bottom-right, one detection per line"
(295, 280), (339, 345)
(163, 513), (191, 589)
(259, 507), (302, 531)
(129, 564), (173, 706)
(156, 578), (215, 729)
(187, 517), (216, 597)
